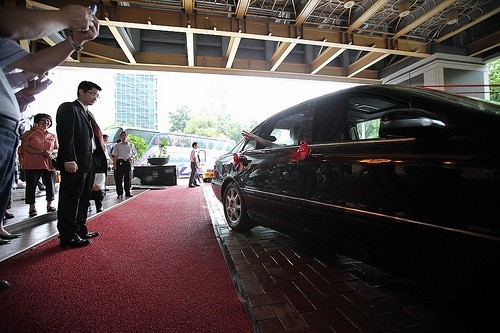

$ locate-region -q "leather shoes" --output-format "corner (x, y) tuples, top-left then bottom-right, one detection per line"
(81, 231), (99, 239)
(60, 233), (89, 250)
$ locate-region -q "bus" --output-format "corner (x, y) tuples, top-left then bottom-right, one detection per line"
(102, 126), (235, 178)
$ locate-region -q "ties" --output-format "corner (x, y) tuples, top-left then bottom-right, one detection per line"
(86, 111), (106, 152)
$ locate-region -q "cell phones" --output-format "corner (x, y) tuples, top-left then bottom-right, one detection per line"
(91, 5), (98, 18)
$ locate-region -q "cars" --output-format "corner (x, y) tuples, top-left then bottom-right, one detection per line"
(210, 83), (500, 295)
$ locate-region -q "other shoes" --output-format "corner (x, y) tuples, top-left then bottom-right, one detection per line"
(97, 205), (102, 212)
(87, 206), (92, 212)
(126, 194), (132, 197)
(0, 280), (10, 289)
(4, 209), (14, 219)
(118, 194), (122, 198)
(194, 183), (200, 186)
(189, 184), (195, 187)
(0, 237), (10, 244)
(0, 232), (21, 238)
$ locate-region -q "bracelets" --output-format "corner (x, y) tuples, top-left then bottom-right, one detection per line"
(68, 35), (84, 52)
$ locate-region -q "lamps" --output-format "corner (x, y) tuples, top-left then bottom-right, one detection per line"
(398, 0), (410, 17)
(446, 8), (458, 25)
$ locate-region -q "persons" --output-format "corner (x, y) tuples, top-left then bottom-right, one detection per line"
(241, 125), (300, 148)
(0, 0), (99, 290)
(55, 81), (107, 248)
(188, 142), (201, 187)
(92, 131), (137, 212)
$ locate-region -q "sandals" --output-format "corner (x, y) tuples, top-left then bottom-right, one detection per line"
(47, 206), (56, 212)
(29, 209), (37, 216)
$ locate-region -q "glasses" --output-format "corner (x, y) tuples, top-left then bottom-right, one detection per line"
(83, 89), (100, 100)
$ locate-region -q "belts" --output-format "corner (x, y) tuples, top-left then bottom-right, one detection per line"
(0, 117), (24, 137)
